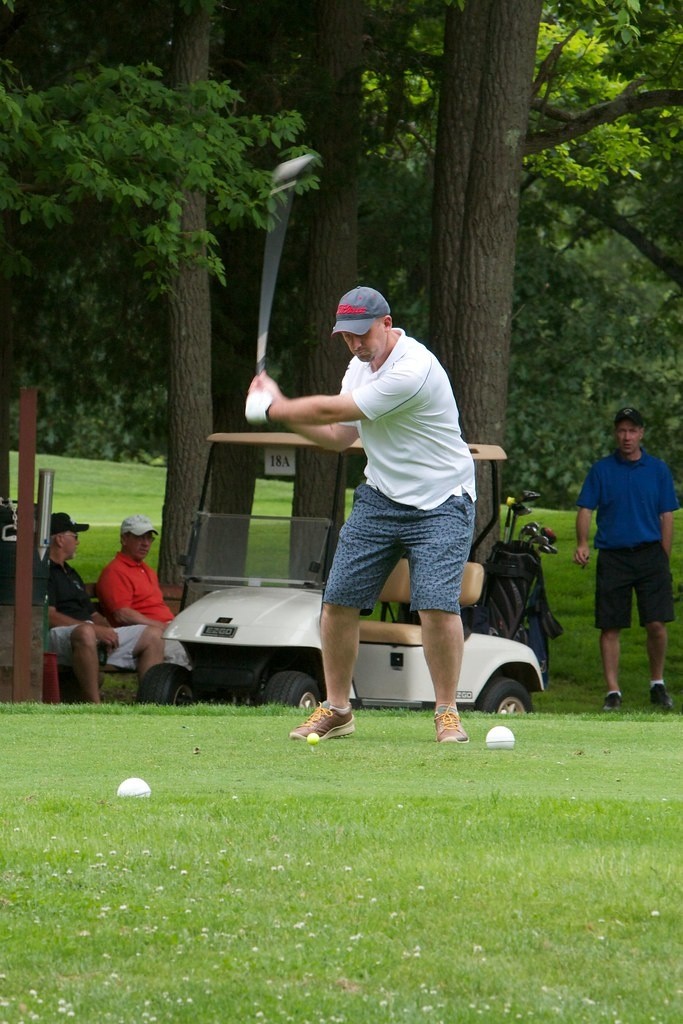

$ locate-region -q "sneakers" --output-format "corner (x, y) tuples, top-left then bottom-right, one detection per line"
(289, 701), (355, 741)
(603, 693), (621, 711)
(649, 683), (672, 710)
(434, 703), (469, 744)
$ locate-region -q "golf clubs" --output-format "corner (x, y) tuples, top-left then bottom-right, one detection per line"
(256, 153), (315, 375)
(505, 490), (558, 556)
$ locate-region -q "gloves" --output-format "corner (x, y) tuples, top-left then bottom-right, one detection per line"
(245, 391), (273, 424)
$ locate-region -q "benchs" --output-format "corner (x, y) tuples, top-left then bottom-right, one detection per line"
(42, 583), (182, 703)
(358, 557), (484, 645)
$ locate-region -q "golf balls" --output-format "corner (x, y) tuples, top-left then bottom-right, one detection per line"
(307, 733), (320, 745)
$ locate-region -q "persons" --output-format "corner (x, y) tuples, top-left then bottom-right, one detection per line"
(40, 513), (165, 703)
(246, 287), (476, 746)
(573, 409), (678, 713)
(97, 514), (193, 670)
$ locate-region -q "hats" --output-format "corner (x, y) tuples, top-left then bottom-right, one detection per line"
(615, 407), (644, 428)
(50, 512), (89, 535)
(121, 514), (158, 537)
(331, 286), (390, 338)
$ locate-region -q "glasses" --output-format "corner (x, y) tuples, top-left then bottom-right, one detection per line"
(63, 534), (78, 540)
(126, 535), (155, 542)
